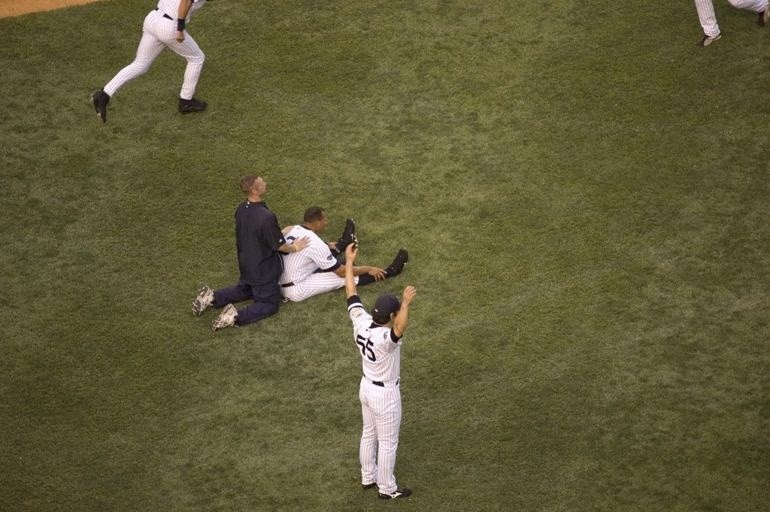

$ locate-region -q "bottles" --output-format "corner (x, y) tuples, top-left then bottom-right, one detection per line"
(374, 295), (400, 312)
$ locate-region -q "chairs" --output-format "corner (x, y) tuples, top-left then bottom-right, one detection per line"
(191, 287), (213, 316)
(699, 33), (721, 47)
(179, 97), (207, 114)
(389, 248), (408, 274)
(340, 217), (357, 247)
(92, 90), (110, 123)
(757, 0), (770, 25)
(211, 304), (238, 332)
(363, 483), (411, 498)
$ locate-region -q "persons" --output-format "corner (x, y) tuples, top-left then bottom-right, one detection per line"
(92, 0), (206, 123)
(191, 174), (312, 331)
(695, 0), (770, 50)
(277, 205), (408, 304)
(345, 242), (417, 499)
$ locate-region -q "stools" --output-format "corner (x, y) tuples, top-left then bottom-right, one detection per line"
(363, 374), (398, 387)
(155, 6), (173, 20)
(281, 282), (294, 287)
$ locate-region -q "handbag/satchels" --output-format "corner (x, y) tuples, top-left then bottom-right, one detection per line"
(176, 18), (186, 32)
